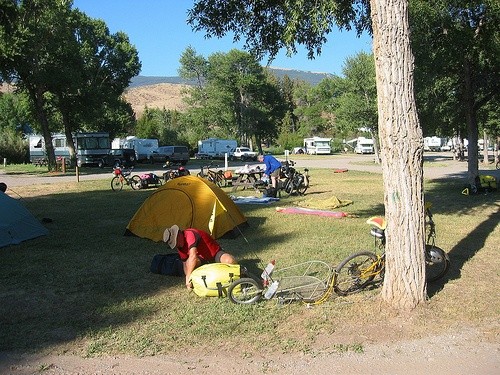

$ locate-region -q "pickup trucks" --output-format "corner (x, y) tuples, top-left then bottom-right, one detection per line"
(229, 147), (258, 161)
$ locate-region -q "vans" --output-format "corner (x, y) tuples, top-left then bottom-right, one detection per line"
(152, 145), (190, 165)
(352, 137), (374, 155)
(111, 136), (158, 160)
(197, 138), (238, 160)
(304, 136), (332, 154)
(423, 136), (493, 151)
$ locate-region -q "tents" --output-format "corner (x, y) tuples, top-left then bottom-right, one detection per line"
(126, 175), (248, 241)
(0, 188), (51, 247)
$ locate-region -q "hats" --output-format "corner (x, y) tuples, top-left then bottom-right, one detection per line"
(162, 224), (179, 250)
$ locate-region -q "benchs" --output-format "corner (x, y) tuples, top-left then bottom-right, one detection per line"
(232, 178), (264, 193)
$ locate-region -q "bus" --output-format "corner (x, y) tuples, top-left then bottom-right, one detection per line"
(29, 132), (111, 168)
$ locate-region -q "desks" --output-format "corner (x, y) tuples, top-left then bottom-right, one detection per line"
(235, 170), (265, 193)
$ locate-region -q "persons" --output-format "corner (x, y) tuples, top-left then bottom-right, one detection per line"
(163, 224), (235, 292)
(0, 182), (7, 192)
(258, 155), (282, 188)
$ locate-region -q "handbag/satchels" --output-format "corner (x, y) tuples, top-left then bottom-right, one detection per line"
(151, 254), (182, 276)
(264, 188), (277, 198)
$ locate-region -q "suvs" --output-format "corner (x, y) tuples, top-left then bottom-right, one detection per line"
(109, 148), (137, 168)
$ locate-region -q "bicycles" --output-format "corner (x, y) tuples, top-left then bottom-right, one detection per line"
(161, 161), (227, 188)
(279, 168), (311, 198)
(496, 155), (500, 169)
(330, 208), (450, 295)
(110, 167), (143, 192)
